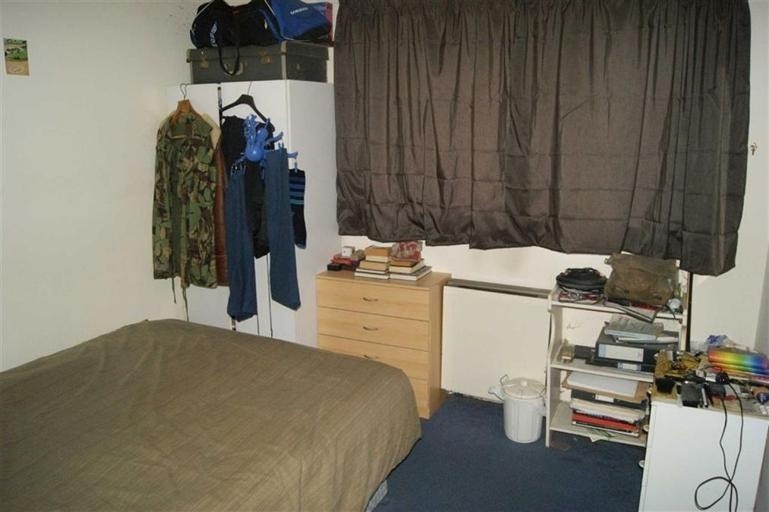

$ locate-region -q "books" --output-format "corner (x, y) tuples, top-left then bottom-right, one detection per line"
(618, 331), (679, 344)
(603, 313), (666, 340)
(353, 245), (433, 281)
(559, 370), (650, 439)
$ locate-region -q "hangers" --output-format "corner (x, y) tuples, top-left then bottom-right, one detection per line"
(158, 81), (277, 138)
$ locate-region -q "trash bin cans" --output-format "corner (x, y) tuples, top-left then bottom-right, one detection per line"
(500, 375), (545, 443)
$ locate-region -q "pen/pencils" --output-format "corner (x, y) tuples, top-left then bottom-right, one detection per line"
(701, 387), (708, 408)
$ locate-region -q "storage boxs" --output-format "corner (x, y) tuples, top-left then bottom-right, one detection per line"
(185, 38), (329, 84)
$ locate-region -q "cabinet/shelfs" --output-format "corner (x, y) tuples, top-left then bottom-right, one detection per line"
(314, 272), (451, 420)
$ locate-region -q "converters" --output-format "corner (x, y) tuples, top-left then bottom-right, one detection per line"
(710, 382), (725, 396)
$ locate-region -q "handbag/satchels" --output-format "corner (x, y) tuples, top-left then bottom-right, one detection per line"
(189, 1), (332, 76)
(602, 250), (680, 310)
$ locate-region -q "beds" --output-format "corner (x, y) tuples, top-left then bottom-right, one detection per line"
(1, 317), (421, 512)
